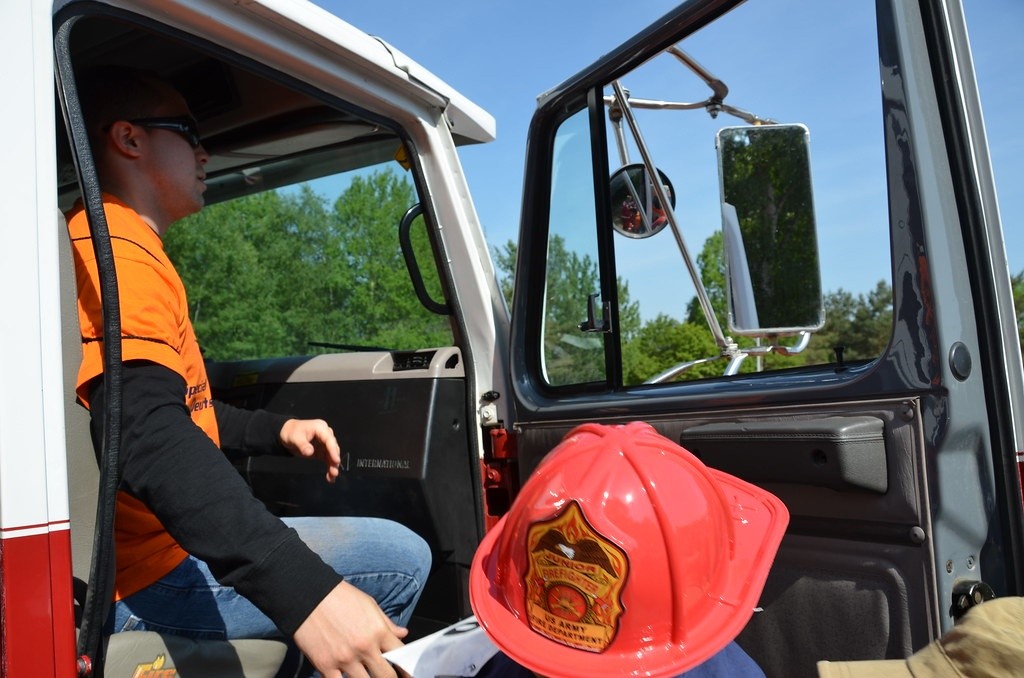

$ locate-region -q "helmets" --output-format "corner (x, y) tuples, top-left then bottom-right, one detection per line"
(469, 421), (789, 678)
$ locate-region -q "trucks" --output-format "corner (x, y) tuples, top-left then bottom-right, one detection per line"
(0, 0), (1024, 678)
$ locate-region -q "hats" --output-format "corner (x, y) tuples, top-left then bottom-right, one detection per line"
(817, 596), (1024, 678)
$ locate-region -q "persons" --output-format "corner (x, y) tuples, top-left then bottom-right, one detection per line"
(622, 195), (634, 231)
(468, 422), (1024, 678)
(67, 62), (433, 678)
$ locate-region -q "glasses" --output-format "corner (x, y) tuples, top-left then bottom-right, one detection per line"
(101, 113), (200, 149)
